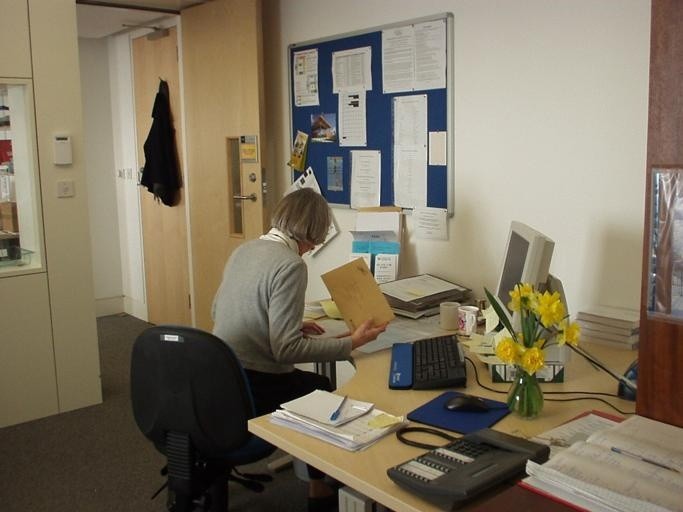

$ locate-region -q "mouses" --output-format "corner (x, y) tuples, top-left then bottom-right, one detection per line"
(445, 394), (487, 414)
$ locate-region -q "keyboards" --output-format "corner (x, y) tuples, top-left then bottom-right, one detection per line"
(412, 332), (468, 389)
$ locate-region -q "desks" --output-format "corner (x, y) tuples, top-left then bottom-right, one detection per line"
(252, 301), (633, 511)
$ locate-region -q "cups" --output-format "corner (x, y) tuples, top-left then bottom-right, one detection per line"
(457, 305), (480, 337)
(438, 301), (461, 330)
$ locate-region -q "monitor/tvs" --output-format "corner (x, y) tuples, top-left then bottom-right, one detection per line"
(493, 220), (570, 363)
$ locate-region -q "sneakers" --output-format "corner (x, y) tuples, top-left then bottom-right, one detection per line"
(307, 483), (344, 512)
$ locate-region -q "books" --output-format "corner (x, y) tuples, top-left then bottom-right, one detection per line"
(573, 305), (640, 350)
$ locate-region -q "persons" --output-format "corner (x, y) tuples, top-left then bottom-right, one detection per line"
(211, 189), (389, 509)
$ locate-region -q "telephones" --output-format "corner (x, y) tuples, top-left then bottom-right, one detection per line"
(386, 429), (550, 511)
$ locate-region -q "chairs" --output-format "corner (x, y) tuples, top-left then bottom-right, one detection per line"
(123, 320), (283, 512)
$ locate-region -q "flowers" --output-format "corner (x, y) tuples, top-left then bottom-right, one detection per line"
(481, 274), (587, 376)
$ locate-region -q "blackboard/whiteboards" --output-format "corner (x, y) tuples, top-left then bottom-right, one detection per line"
(285, 15), (457, 218)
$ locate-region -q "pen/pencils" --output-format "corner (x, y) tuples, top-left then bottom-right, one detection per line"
(330, 394), (348, 421)
(611, 447), (681, 474)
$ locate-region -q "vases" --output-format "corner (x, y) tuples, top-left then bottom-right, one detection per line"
(508, 359), (544, 420)
